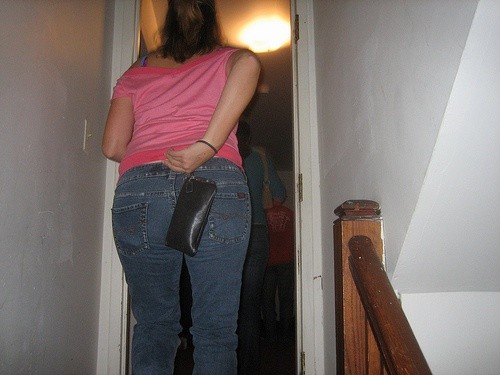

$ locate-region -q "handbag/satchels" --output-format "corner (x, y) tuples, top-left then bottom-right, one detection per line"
(258, 148), (274, 210)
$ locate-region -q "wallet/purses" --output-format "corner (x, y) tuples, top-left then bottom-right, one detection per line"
(163, 177), (218, 259)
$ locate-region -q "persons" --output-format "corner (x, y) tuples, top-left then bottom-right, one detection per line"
(237, 121), (295, 374)
(102, 0), (263, 375)
